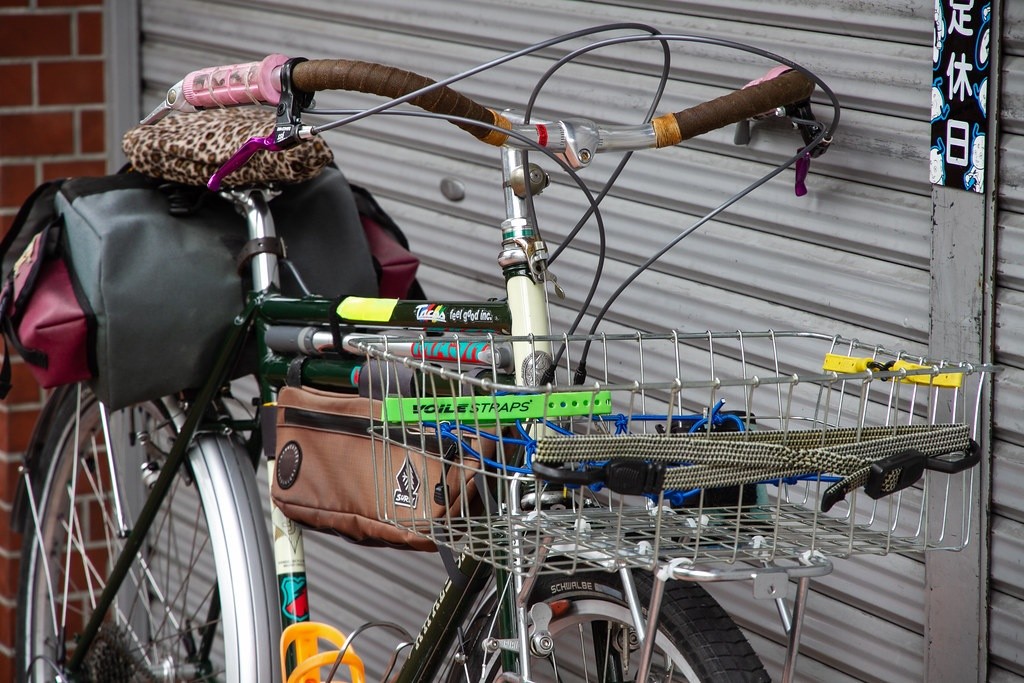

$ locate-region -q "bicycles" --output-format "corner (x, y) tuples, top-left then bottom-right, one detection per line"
(9, 22), (1005, 683)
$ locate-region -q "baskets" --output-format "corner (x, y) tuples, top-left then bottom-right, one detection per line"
(344, 328), (1006, 578)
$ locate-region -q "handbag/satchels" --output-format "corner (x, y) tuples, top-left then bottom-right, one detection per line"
(0, 161), (430, 404)
